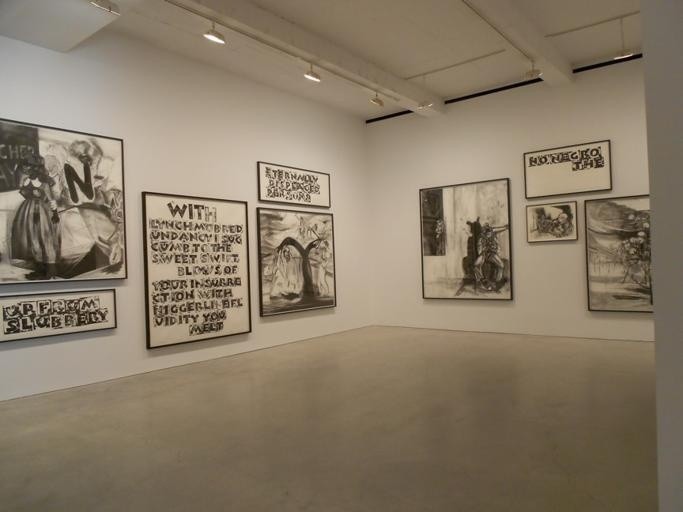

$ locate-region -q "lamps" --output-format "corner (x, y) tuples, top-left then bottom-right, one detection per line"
(88, 1), (386, 107)
(524, 60), (544, 76)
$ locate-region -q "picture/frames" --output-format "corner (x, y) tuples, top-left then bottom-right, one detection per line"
(521, 138), (614, 199)
(419, 177), (515, 302)
(525, 200), (580, 244)
(255, 205), (336, 319)
(1, 287), (119, 342)
(255, 158), (334, 209)
(138, 190), (256, 352)
(582, 194), (651, 312)
(1, 117), (133, 286)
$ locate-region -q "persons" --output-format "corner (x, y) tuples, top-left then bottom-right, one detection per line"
(11, 154), (60, 280)
(473, 222), (508, 287)
